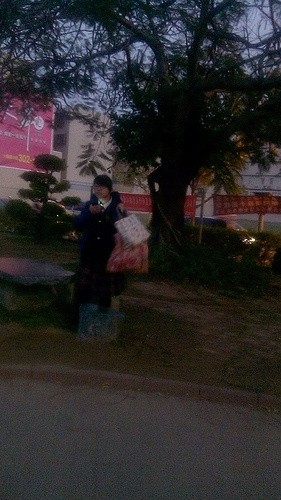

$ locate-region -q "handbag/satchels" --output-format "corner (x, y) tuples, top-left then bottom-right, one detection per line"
(115, 204), (149, 246)
(105, 232), (149, 273)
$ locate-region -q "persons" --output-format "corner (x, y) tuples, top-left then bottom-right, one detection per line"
(68, 174), (134, 314)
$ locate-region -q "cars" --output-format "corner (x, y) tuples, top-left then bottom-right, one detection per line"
(184, 217), (256, 255)
